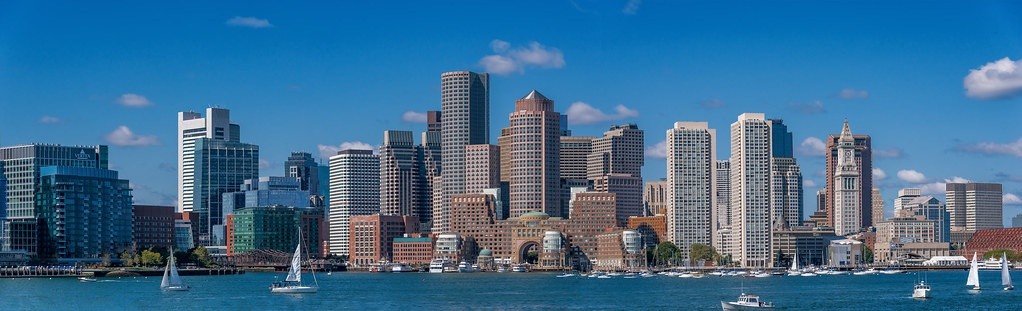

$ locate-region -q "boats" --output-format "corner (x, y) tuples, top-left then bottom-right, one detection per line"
(721, 279), (776, 311)
(912, 270), (931, 298)
(78, 276), (96, 282)
(512, 263), (526, 272)
(369, 261), (412, 272)
(427, 257), (478, 274)
(497, 266), (507, 272)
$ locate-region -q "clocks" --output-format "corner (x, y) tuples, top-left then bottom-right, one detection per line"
(845, 151), (851, 156)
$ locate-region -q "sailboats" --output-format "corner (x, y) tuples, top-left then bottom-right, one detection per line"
(160, 246), (192, 291)
(965, 252), (982, 294)
(1001, 251), (1014, 291)
(269, 226), (320, 293)
(556, 243), (907, 279)
